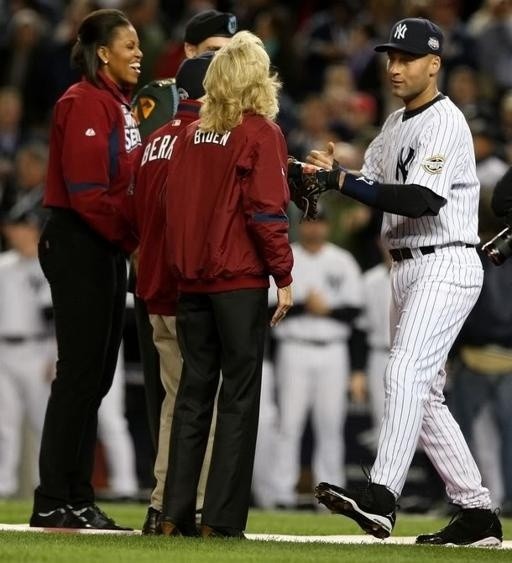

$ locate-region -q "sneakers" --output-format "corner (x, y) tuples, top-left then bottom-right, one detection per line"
(415, 507), (503, 548)
(28, 506), (137, 530)
(315, 479), (398, 539)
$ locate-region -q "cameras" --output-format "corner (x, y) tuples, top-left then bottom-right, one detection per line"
(481, 204), (512, 267)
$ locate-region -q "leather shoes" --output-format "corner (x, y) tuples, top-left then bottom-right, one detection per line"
(140, 506), (164, 535)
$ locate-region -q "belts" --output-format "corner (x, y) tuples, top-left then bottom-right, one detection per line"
(1, 332), (55, 345)
(389, 246), (436, 262)
(298, 338), (337, 346)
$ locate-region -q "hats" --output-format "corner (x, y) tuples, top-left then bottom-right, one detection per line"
(175, 51), (217, 98)
(183, 10), (239, 45)
(373, 17), (443, 58)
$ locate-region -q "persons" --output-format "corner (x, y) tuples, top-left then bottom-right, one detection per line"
(281, 18), (500, 547)
(24, 7), (141, 530)
(2, 3), (509, 518)
(157, 26), (295, 537)
(135, 50), (213, 540)
